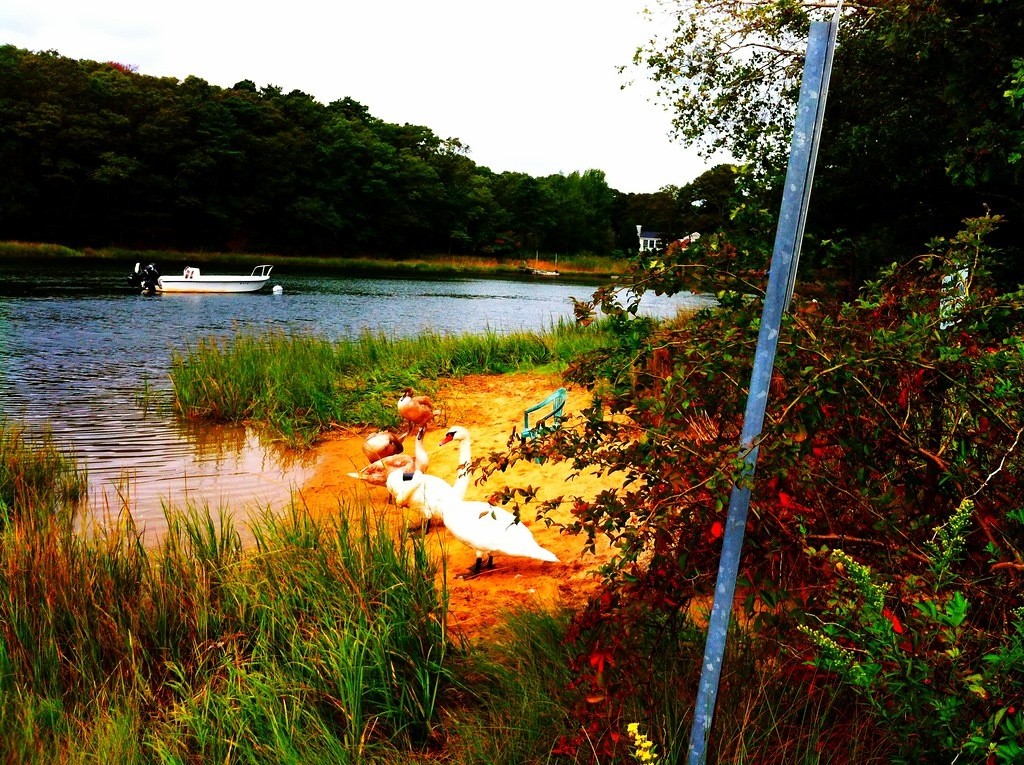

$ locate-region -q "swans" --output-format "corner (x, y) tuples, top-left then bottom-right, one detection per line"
(386, 426), (562, 583)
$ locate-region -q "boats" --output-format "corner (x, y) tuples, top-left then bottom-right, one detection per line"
(142, 265), (274, 293)
(533, 270), (560, 276)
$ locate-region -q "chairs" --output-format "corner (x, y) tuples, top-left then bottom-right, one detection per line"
(521, 388), (570, 464)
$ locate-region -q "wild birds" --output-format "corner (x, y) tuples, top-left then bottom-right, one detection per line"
(347, 387), (442, 506)
